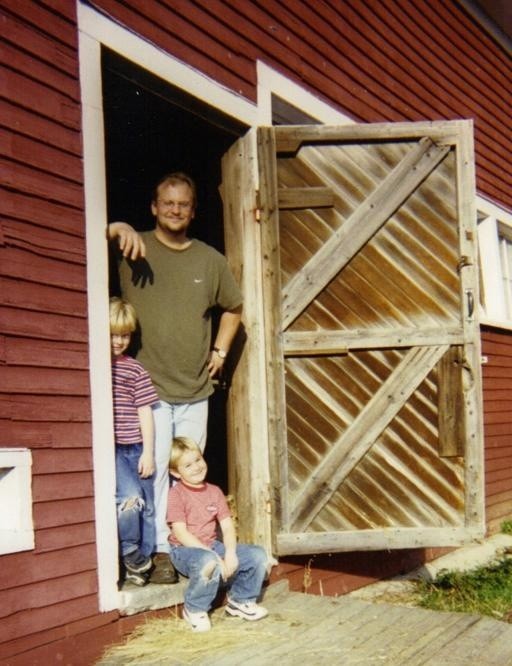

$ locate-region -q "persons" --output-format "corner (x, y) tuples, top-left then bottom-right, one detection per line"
(109, 296), (159, 583)
(167, 434), (273, 632)
(104, 172), (243, 583)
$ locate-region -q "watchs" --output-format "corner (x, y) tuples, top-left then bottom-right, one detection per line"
(212, 345), (228, 360)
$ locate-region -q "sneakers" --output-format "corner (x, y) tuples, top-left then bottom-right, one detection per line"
(121, 550), (178, 586)
(182, 605), (210, 631)
(224, 595), (270, 622)
